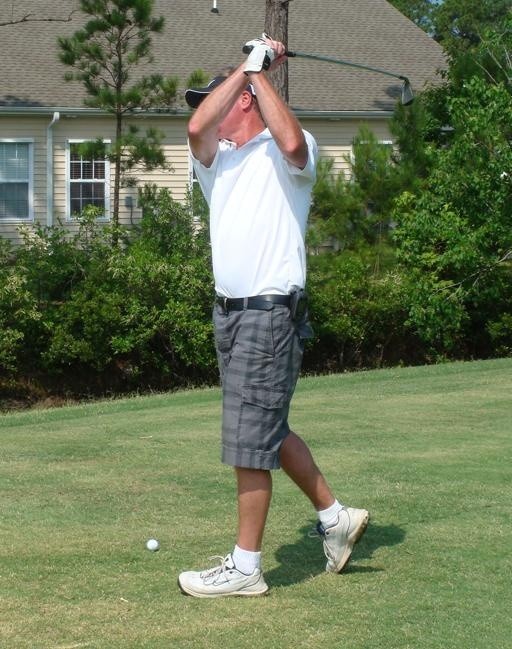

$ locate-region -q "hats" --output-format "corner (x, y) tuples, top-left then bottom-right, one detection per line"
(184, 76), (257, 109)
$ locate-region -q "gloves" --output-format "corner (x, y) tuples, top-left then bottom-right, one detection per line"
(243, 32), (276, 75)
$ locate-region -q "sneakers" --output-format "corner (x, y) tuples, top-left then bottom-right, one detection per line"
(177, 553), (269, 599)
(308, 506), (370, 574)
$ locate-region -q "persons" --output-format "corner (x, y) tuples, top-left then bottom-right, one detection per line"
(178, 33), (370, 599)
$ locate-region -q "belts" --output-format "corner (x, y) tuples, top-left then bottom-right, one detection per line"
(215, 294), (290, 311)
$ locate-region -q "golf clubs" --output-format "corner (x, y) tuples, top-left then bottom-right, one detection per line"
(243, 46), (414, 106)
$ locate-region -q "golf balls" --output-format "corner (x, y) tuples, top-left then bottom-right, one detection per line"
(147, 539), (158, 551)
